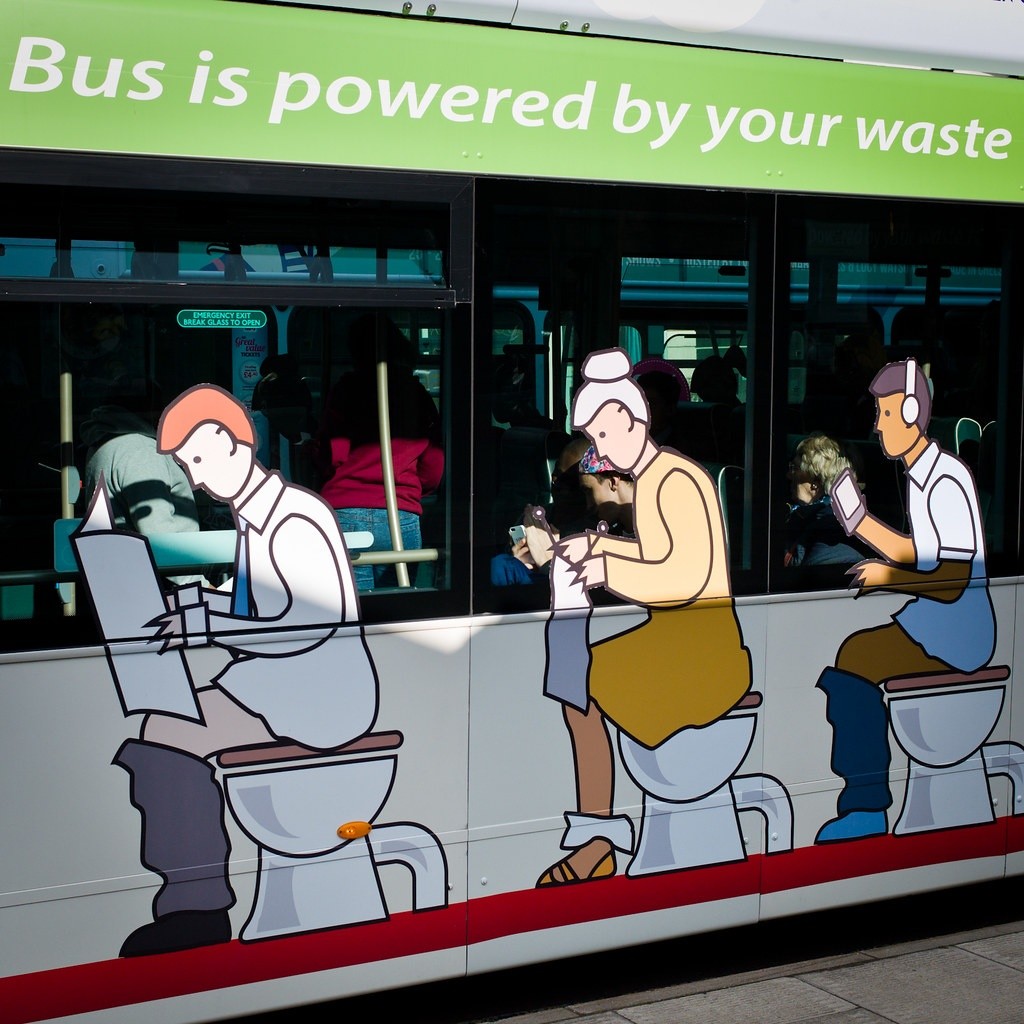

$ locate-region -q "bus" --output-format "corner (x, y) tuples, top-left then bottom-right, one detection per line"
(1, 0), (1024, 1024)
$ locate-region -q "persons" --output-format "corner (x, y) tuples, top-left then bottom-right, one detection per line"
(77, 317), (871, 595)
